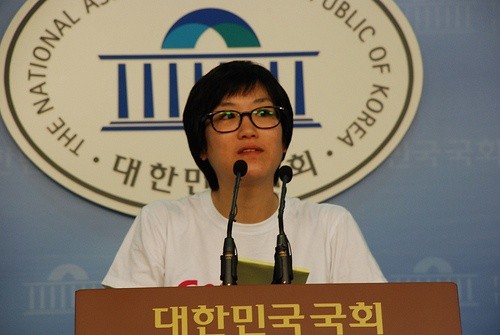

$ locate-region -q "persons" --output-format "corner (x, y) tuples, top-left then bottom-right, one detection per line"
(101, 60), (389, 289)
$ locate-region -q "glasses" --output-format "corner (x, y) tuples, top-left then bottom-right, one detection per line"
(208, 106), (285, 133)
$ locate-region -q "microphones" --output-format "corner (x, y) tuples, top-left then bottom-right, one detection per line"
(271, 166), (293, 285)
(220, 160), (248, 285)
